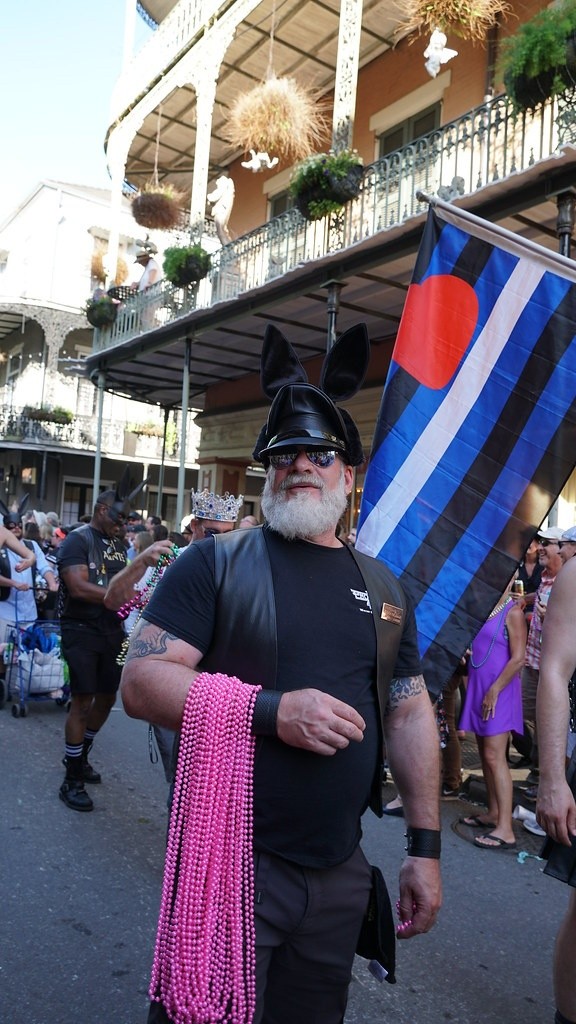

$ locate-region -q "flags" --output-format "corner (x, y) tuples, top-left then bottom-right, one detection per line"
(357, 205), (576, 698)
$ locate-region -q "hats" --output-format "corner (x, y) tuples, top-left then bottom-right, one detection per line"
(558, 526), (576, 543)
(128, 512), (141, 520)
(537, 526), (566, 542)
(180, 513), (196, 533)
(252, 382), (366, 468)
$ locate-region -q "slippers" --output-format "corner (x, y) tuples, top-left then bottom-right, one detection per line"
(458, 814), (497, 828)
(472, 833), (517, 849)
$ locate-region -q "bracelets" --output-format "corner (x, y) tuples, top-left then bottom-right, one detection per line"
(249, 689), (284, 739)
(403, 825), (443, 859)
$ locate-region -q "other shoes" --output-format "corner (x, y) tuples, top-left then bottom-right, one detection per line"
(524, 784), (538, 800)
(438, 782), (461, 801)
(383, 800), (404, 815)
(523, 819), (547, 837)
(519, 768), (541, 791)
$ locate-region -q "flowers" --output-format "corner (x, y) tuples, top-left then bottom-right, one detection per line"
(124, 419), (178, 456)
(285, 148), (364, 220)
(80, 289), (114, 312)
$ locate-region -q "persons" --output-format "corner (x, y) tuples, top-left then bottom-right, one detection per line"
(0, 514), (59, 701)
(136, 233), (158, 253)
(422, 26), (457, 76)
(56, 485), (130, 811)
(348, 522), (576, 850)
(206, 176), (235, 247)
(127, 248), (162, 330)
(535, 554), (576, 1024)
(0, 486), (258, 634)
(102, 486), (244, 778)
(241, 149), (279, 174)
(118, 383), (445, 1024)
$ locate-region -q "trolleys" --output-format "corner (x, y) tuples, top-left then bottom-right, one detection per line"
(0, 587), (73, 718)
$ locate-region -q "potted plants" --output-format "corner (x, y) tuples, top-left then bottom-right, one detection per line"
(162, 242), (213, 288)
(485, 0), (576, 124)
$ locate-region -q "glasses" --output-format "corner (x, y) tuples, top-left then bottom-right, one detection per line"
(127, 518), (138, 523)
(538, 540), (558, 547)
(265, 449), (348, 469)
(557, 539), (576, 550)
(197, 521), (232, 538)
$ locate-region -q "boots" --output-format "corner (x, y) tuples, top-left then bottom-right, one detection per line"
(80, 735), (101, 783)
(58, 756), (93, 811)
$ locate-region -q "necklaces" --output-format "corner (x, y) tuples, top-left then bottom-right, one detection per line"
(150, 673), (262, 1024)
(470, 597), (507, 668)
(114, 545), (180, 666)
(487, 596), (512, 619)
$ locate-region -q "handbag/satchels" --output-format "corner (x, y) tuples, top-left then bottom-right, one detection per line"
(0, 622), (72, 705)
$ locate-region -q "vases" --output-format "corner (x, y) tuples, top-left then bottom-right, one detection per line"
(87, 304), (118, 328)
(296, 165), (364, 220)
(124, 431), (168, 458)
